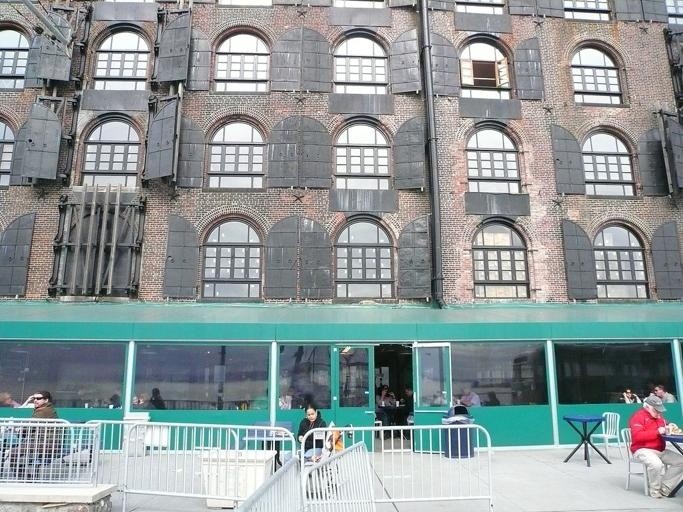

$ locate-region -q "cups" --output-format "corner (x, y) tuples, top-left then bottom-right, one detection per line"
(664, 426), (672, 436)
(456, 399), (460, 404)
(395, 401), (400, 407)
(108, 404), (114, 409)
(85, 403), (88, 408)
(381, 401), (385, 406)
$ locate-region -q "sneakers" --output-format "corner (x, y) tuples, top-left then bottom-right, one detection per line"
(649, 490), (663, 499)
(662, 487), (678, 497)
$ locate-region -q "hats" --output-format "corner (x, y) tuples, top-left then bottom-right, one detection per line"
(643, 394), (667, 414)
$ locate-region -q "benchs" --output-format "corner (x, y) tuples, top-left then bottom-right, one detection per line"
(1, 420), (102, 479)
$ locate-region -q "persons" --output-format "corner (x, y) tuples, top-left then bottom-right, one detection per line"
(376, 384), (397, 440)
(19, 394), (33, 408)
(653, 384), (678, 403)
(110, 394), (120, 407)
(286, 385), (302, 409)
(0, 389), (21, 408)
(399, 386), (413, 440)
(9, 390), (61, 481)
(298, 402), (329, 463)
(148, 388), (166, 410)
(458, 383), (482, 407)
(629, 396), (682, 499)
(621, 388), (641, 403)
(486, 392), (500, 405)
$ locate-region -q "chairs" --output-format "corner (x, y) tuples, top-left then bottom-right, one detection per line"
(619, 425), (668, 498)
(587, 410), (626, 465)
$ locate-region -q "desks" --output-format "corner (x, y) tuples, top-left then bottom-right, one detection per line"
(378, 404), (410, 443)
(558, 411), (614, 469)
(659, 429), (683, 499)
(241, 419), (294, 475)
(197, 445), (278, 509)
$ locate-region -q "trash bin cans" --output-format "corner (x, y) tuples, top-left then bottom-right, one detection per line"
(443, 406), (475, 458)
(123, 412), (152, 457)
